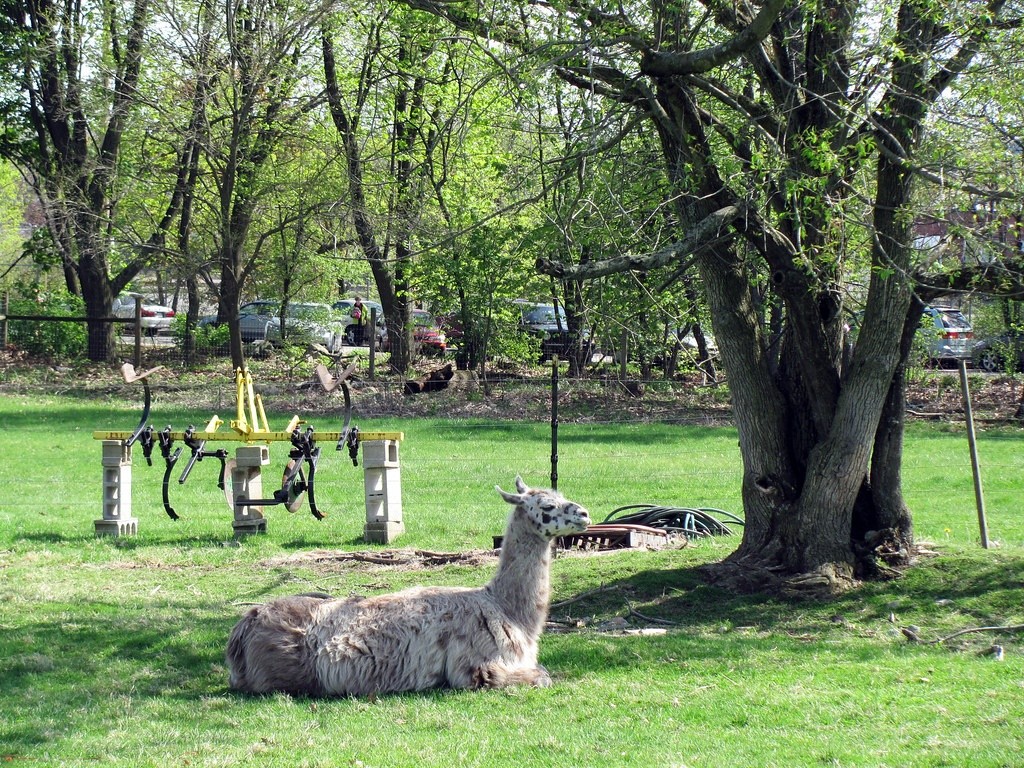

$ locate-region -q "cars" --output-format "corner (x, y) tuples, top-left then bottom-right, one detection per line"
(600, 312), (722, 370)
(203, 302), (343, 353)
(331, 300), (385, 345)
(377, 308), (447, 354)
(112, 291), (177, 336)
(436, 309), (502, 341)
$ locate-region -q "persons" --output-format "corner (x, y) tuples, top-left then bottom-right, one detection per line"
(348, 297), (368, 348)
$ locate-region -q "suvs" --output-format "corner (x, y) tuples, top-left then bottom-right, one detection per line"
(849, 304), (975, 368)
(487, 298), (595, 365)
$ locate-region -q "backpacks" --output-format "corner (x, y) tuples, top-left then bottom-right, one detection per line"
(351, 307), (362, 319)
